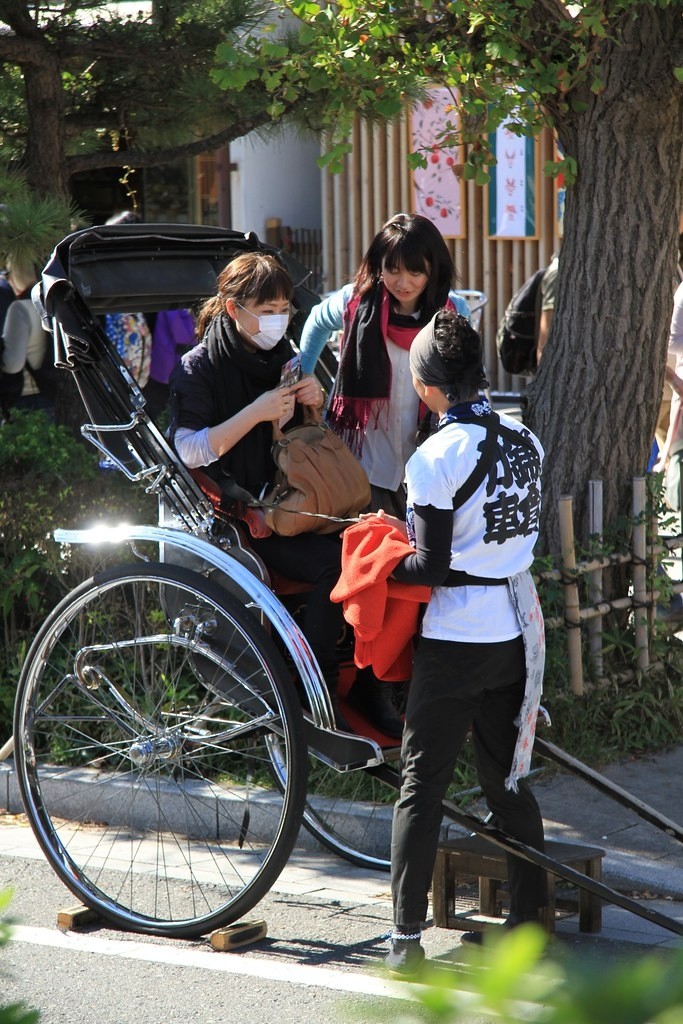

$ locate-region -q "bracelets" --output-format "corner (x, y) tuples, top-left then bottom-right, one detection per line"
(315, 387), (327, 416)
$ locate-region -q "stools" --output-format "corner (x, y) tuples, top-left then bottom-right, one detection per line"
(431, 836), (605, 936)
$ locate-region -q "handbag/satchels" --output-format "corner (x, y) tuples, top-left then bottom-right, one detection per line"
(264, 393), (371, 536)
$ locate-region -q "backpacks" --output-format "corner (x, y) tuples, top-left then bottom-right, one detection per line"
(495, 269), (538, 377)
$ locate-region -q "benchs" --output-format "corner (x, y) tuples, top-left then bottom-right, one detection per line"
(193, 472), (310, 606)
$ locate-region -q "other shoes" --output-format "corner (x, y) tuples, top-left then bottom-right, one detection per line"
(346, 683), (405, 739)
(297, 673), (355, 735)
(390, 682), (410, 714)
(383, 929), (424, 978)
(460, 914), (548, 958)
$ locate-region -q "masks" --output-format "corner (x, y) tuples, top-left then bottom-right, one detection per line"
(236, 303), (290, 351)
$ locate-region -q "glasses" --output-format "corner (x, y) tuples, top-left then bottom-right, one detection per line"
(1, 271), (12, 280)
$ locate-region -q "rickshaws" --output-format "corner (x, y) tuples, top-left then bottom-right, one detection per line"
(9, 217), (683, 942)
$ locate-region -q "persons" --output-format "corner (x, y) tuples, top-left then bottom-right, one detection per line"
(0, 252), (47, 410)
(536, 261), (559, 363)
(298, 214), (472, 522)
(169, 253), (408, 749)
(653, 232), (683, 532)
(327, 311), (546, 976)
